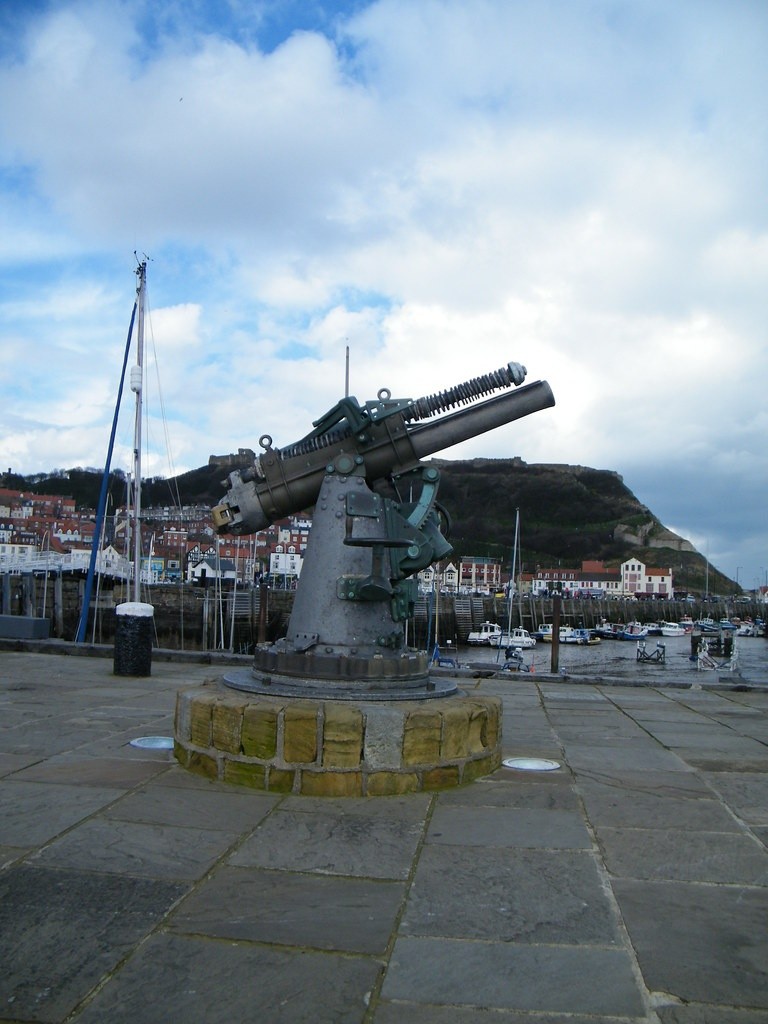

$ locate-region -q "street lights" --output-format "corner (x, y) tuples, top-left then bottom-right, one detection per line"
(737, 566), (743, 601)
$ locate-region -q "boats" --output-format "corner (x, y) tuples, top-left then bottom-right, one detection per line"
(468, 621), (502, 646)
(531, 616), (766, 645)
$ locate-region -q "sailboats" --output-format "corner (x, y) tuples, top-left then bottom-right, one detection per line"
(497, 507), (537, 649)
